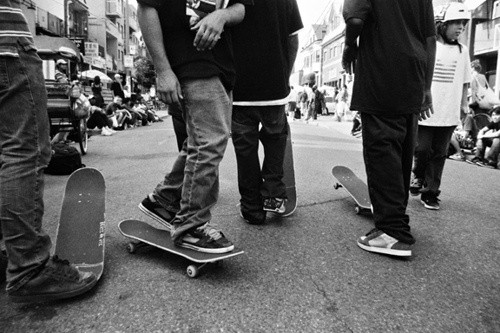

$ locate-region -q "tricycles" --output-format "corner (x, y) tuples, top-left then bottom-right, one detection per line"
(33, 36), (88, 155)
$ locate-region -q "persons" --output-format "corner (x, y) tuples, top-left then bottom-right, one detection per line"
(410, 2), (471, 211)
(55, 59), (79, 89)
(169, 100), (188, 149)
(309, 86), (325, 119)
(448, 101), (484, 160)
(230, 0), (303, 225)
(91, 76), (104, 107)
(136, 0), (254, 254)
(334, 84), (348, 121)
(85, 95), (117, 136)
(0, 0), (98, 303)
(110, 73), (124, 97)
(303, 83), (315, 120)
(340, 0), (437, 258)
(466, 106), (500, 168)
(106, 94), (164, 130)
(288, 85), (297, 124)
(468, 59), (486, 102)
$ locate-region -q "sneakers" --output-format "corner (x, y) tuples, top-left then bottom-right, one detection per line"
(262, 197), (286, 213)
(137, 192), (177, 229)
(356, 227), (412, 257)
(8, 254), (96, 303)
(465, 157), (482, 164)
(106, 126), (116, 134)
(476, 160), (497, 169)
(409, 177), (423, 195)
(449, 152), (464, 159)
(176, 220), (234, 254)
(100, 127), (112, 136)
(420, 197), (440, 210)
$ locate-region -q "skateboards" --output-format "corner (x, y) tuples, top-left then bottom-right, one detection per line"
(117, 218), (244, 278)
(271, 121), (298, 218)
(331, 165), (372, 214)
(53, 167), (106, 282)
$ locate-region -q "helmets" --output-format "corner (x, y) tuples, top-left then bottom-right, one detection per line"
(434, 2), (471, 24)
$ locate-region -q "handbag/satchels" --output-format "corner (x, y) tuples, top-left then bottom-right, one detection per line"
(64, 83), (81, 98)
(43, 142), (85, 176)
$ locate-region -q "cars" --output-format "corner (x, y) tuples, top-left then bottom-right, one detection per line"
(322, 96), (336, 115)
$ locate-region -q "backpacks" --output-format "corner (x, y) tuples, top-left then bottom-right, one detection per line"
(72, 95), (91, 119)
(476, 78), (499, 110)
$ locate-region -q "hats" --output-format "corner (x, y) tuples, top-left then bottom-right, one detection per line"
(55, 59), (68, 64)
